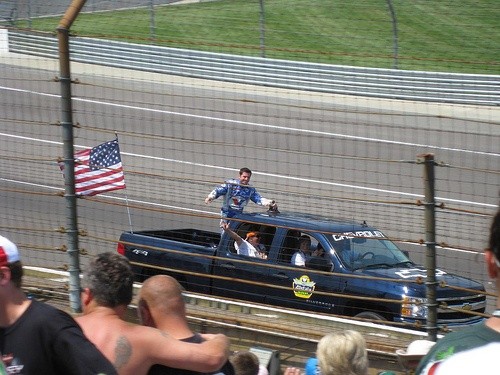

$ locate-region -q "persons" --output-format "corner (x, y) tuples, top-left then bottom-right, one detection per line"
(284, 329), (370, 375)
(411, 210), (500, 375)
(65, 251), (231, 375)
(204, 168), (276, 239)
(231, 351), (260, 375)
(326, 240), (362, 273)
(219, 220), (267, 260)
(377, 340), (436, 375)
(0, 234), (117, 375)
(138, 274), (236, 375)
(290, 235), (323, 268)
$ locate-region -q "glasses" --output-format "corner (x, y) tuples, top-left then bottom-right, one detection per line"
(252, 234), (261, 238)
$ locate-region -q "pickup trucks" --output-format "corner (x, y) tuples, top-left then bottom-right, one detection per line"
(117, 210), (487, 332)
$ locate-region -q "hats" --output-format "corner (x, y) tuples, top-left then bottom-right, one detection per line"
(396, 340), (437, 370)
(0, 235), (20, 265)
(245, 227), (260, 241)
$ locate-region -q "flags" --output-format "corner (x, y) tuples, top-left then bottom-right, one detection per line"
(57, 138), (126, 198)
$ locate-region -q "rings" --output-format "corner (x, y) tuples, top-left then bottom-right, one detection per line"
(290, 372), (294, 373)
